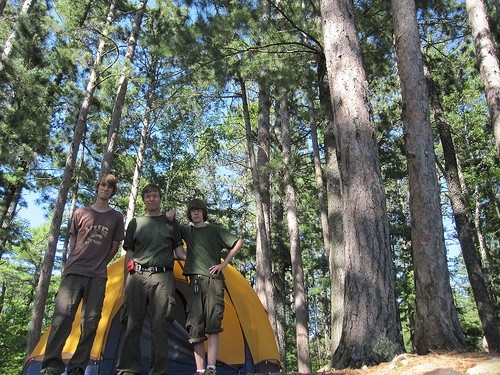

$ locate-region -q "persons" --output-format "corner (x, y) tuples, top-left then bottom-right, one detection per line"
(166, 199), (244, 375)
(114, 184), (187, 375)
(40, 174), (125, 375)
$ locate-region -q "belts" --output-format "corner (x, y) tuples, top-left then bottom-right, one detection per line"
(141, 266), (173, 273)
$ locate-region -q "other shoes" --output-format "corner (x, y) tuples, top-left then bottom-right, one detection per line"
(193, 367), (216, 375)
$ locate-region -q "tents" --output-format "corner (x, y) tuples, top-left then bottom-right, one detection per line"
(18, 239), (282, 375)
